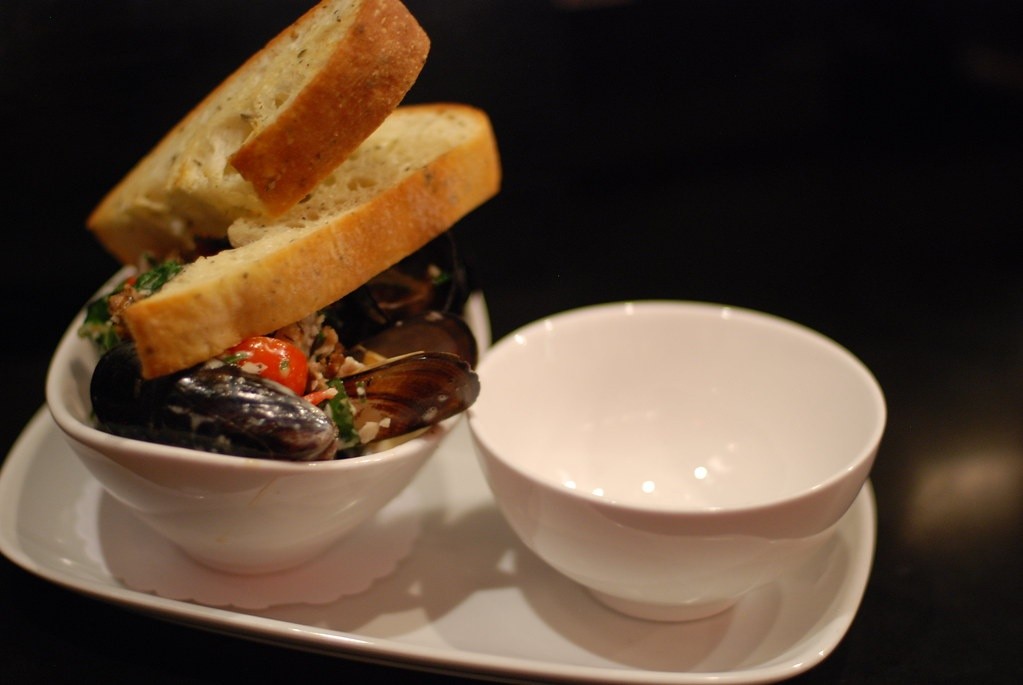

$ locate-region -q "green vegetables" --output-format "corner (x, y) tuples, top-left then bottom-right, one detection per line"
(75, 254), (368, 447)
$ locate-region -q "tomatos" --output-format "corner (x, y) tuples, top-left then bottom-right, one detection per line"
(228, 336), (309, 397)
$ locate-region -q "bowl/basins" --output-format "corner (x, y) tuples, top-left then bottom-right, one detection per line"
(45, 263), (492, 577)
(464, 299), (887, 623)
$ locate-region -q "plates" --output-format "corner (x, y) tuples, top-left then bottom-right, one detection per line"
(2, 402), (878, 685)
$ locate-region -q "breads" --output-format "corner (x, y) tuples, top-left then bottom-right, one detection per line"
(86, 0), (430, 267)
(119, 102), (501, 377)
